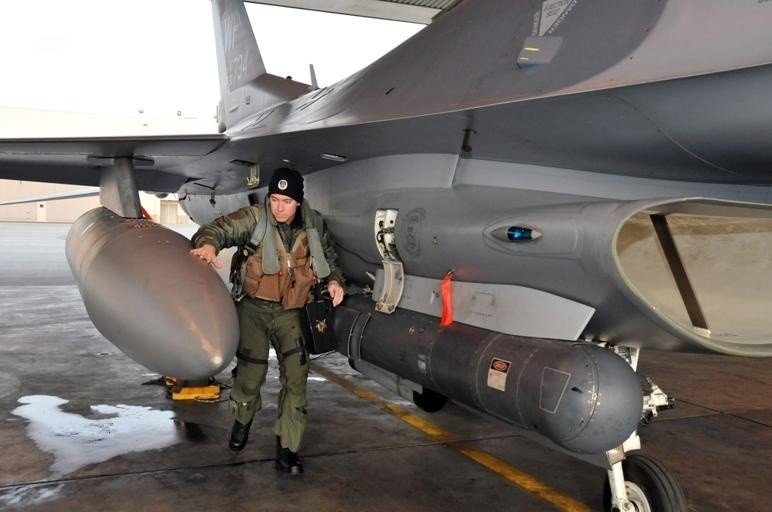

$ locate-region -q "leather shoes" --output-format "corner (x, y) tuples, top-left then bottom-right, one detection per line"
(229, 417), (253, 451)
(275, 437), (304, 475)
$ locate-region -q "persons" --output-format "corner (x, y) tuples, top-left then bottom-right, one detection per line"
(185, 165), (345, 477)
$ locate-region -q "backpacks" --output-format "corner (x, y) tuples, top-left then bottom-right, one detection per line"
(229, 203), (267, 302)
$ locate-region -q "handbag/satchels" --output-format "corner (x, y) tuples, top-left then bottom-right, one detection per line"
(300, 283), (339, 354)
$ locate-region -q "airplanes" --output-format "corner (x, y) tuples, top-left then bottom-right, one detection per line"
(0, 0), (772, 512)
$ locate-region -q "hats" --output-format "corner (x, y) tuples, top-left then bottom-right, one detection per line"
(267, 168), (304, 204)
